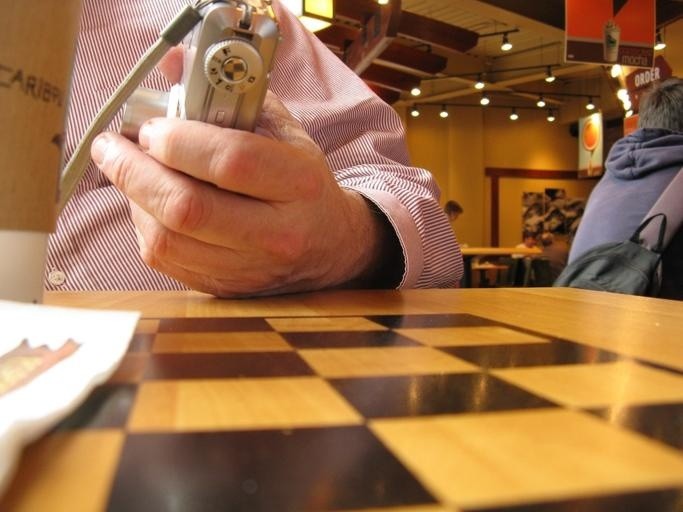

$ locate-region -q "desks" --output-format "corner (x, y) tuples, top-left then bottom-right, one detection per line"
(457, 245), (545, 288)
(0, 284), (682, 512)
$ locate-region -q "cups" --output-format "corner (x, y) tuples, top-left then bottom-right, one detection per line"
(603, 26), (621, 64)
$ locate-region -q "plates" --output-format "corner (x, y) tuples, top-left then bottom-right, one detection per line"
(2, 297), (143, 500)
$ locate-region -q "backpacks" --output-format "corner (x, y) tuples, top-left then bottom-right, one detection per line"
(552, 213), (666, 297)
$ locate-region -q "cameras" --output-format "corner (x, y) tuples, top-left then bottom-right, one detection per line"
(119, 0), (280, 144)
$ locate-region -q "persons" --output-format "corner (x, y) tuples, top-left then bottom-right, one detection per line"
(551, 75), (682, 302)
(444, 200), (464, 222)
(510, 229), (569, 287)
(43, 0), (464, 300)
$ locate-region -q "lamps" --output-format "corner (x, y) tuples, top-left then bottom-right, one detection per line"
(282, 0), (334, 35)
(409, 28), (666, 123)
